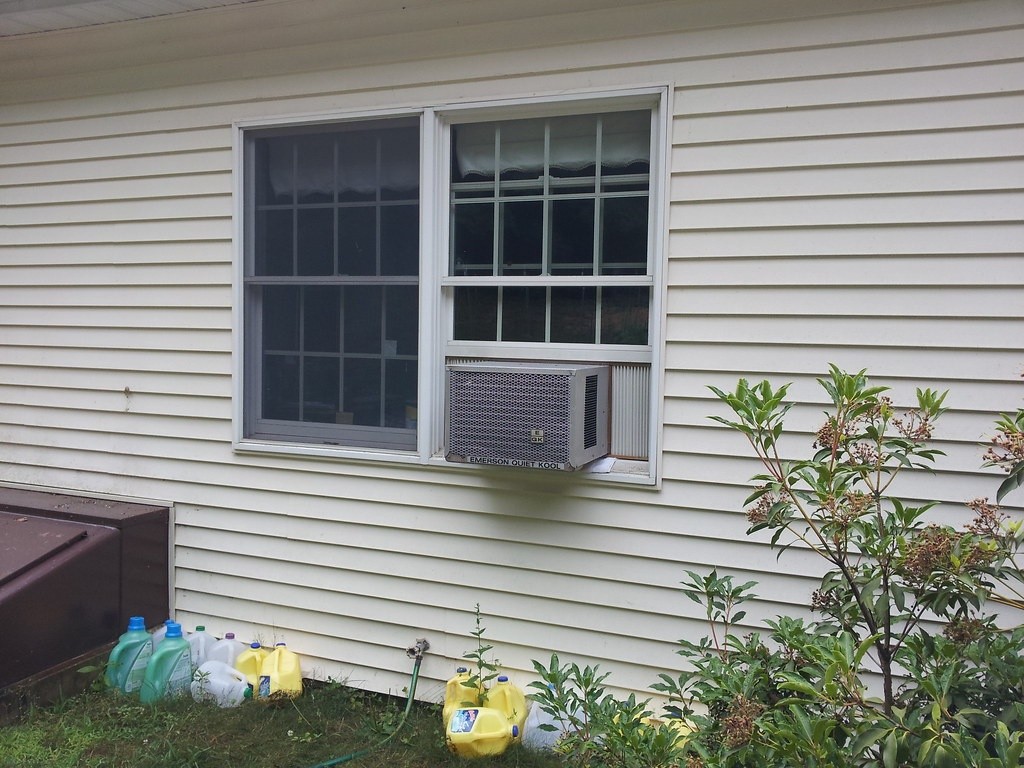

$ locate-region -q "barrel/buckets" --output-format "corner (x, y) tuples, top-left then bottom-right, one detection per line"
(441, 663), (571, 758)
(104, 610), (302, 709)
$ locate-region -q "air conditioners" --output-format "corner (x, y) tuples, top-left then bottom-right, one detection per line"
(443, 361), (610, 473)
